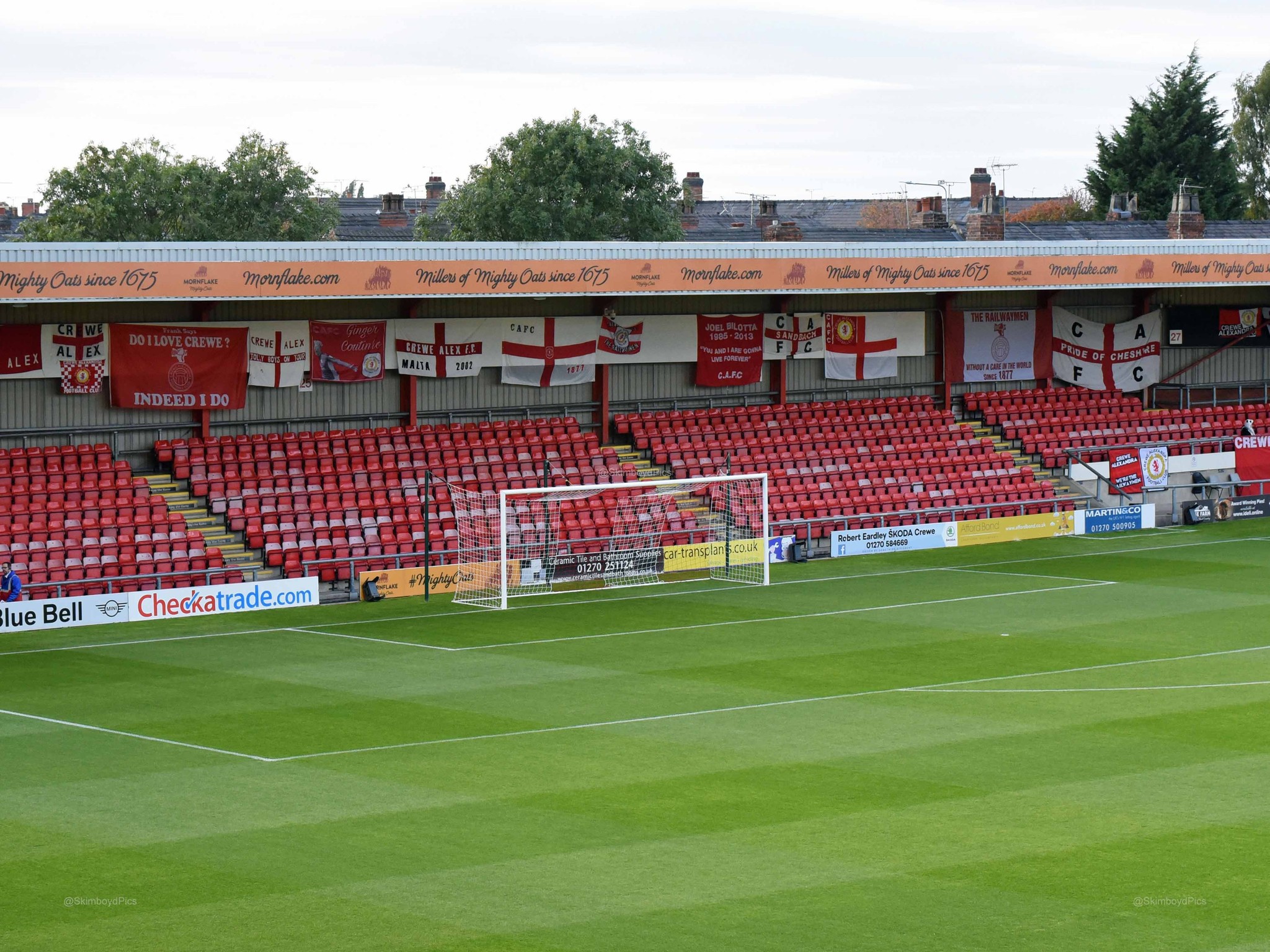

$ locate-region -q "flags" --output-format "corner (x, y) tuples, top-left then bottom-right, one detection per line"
(1052, 306), (1163, 392)
(764, 313), (825, 355)
(501, 317), (596, 387)
(50, 324), (108, 395)
(823, 312), (898, 380)
(1218, 307), (1262, 338)
(598, 315), (645, 355)
(394, 319), (490, 378)
(942, 302), (1053, 383)
(107, 322), (249, 409)
(308, 319), (387, 382)
(247, 327), (309, 389)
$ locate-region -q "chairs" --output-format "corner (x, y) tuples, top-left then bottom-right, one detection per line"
(0, 386), (1270, 603)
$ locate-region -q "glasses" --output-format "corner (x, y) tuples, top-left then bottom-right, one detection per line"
(1, 566), (9, 569)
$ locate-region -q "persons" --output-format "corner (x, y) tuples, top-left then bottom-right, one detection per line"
(0, 561), (22, 603)
(1241, 418), (1258, 436)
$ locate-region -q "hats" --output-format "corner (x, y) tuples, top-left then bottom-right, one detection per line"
(1244, 419), (1255, 436)
